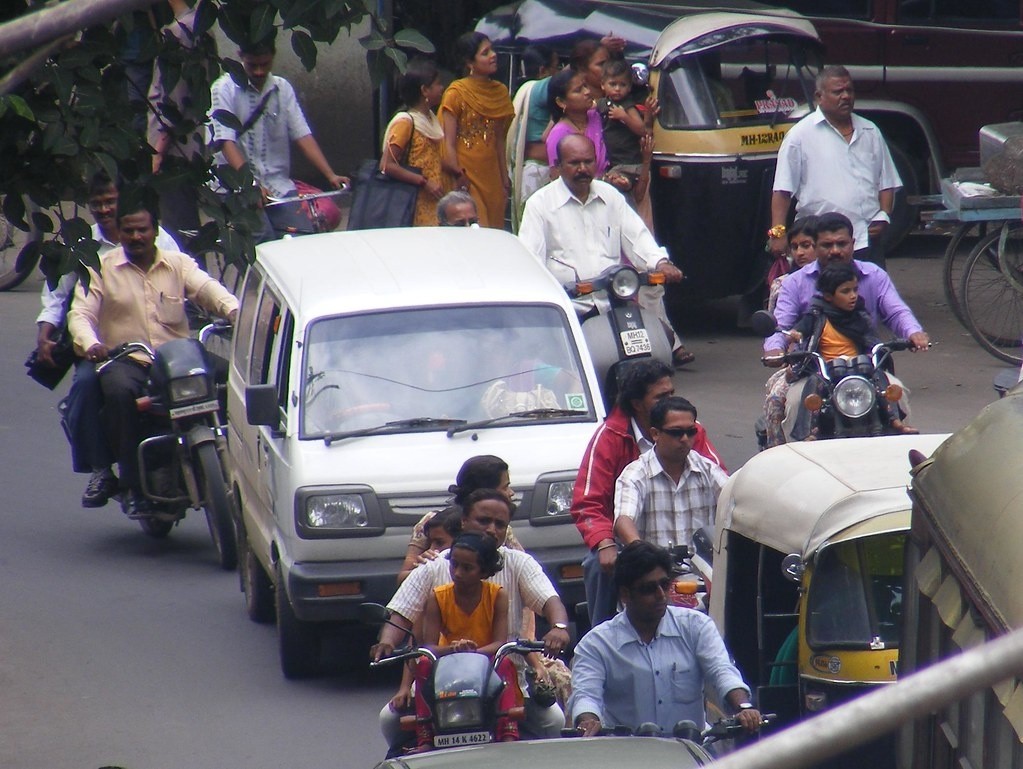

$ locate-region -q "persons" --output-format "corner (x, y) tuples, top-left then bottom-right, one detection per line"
(36, 174), (239, 515)
(764, 213), (931, 450)
(567, 540), (762, 762)
(771, 66), (905, 274)
(380, 31), (696, 367)
(153, 1), (350, 267)
(570, 359), (731, 630)
(369, 455), (573, 758)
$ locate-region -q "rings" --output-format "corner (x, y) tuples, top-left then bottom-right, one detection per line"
(581, 728), (586, 731)
(576, 727), (581, 731)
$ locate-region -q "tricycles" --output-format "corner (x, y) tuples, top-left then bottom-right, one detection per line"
(903, 166), (1022, 367)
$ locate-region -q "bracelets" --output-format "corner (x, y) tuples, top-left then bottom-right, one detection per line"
(765, 223), (786, 240)
(597, 543), (616, 551)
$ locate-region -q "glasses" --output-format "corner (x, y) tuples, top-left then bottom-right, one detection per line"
(445, 218), (477, 226)
(659, 427), (698, 437)
(625, 575), (669, 593)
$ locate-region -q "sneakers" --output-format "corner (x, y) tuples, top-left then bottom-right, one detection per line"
(81, 465), (120, 508)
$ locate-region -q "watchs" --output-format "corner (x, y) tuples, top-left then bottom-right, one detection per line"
(553, 622), (567, 629)
(734, 703), (755, 713)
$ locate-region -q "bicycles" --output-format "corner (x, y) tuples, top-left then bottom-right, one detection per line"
(178, 180), (352, 296)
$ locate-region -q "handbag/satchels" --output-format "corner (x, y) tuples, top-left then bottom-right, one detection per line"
(345, 110), (424, 231)
(23, 313), (76, 391)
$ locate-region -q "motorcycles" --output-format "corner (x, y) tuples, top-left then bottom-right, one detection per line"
(475, 1), (1022, 262)
(96, 316), (239, 574)
(751, 310), (933, 440)
(629, 4), (829, 338)
(705, 433), (956, 768)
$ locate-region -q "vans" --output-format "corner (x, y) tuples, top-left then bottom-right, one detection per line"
(228, 224), (609, 682)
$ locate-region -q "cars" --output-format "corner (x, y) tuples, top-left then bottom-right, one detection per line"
(373, 735), (718, 768)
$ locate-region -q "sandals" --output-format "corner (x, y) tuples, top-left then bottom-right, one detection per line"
(670, 346), (697, 366)
(889, 423), (918, 434)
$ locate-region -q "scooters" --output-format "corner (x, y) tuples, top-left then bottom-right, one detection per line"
(558, 541), (778, 751)
(353, 601), (566, 759)
